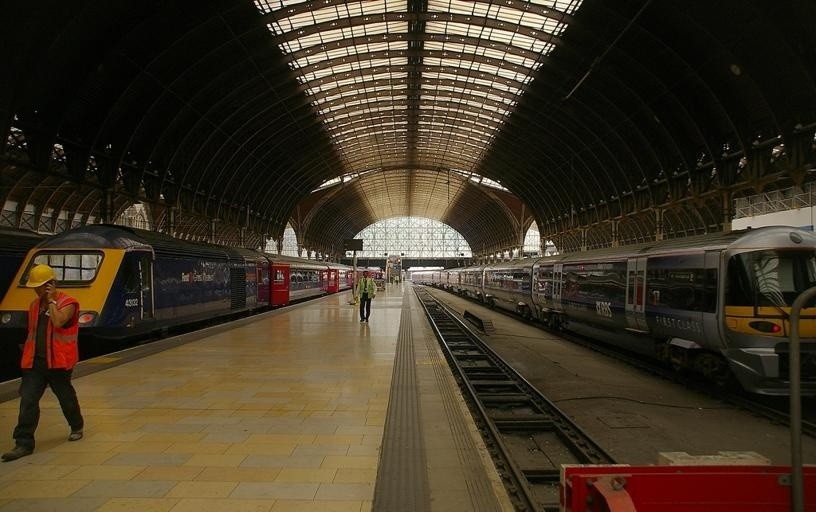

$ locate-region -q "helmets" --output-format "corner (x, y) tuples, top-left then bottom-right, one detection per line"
(26, 264), (56, 287)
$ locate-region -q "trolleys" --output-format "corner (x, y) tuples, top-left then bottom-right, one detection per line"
(373, 279), (385, 291)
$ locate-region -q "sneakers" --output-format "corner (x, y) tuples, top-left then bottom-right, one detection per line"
(1, 446), (33, 460)
(68, 428), (83, 441)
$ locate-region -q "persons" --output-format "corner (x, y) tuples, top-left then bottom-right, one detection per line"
(1, 264), (83, 462)
(390, 275), (393, 284)
(395, 275), (399, 285)
(356, 270), (377, 322)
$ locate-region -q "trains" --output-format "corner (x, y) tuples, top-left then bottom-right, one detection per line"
(1, 222), (385, 352)
(410, 224), (816, 398)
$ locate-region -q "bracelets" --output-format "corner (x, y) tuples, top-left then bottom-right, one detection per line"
(47, 301), (57, 305)
